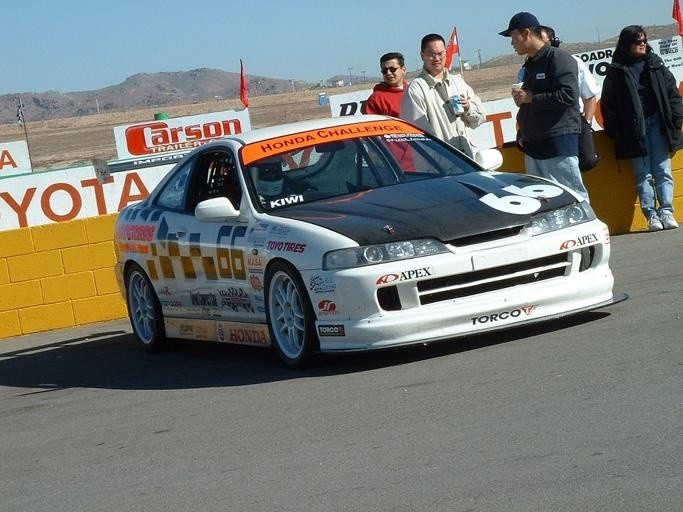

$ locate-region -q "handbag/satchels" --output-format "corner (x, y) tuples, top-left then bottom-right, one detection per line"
(579, 113), (601, 173)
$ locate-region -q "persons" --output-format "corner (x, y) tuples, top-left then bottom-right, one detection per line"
(518, 25), (597, 172)
(601, 25), (683, 230)
(361, 52), (416, 173)
(240, 59), (248, 108)
(402, 34), (485, 160)
(499, 12), (592, 205)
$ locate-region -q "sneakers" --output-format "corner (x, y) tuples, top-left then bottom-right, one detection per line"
(659, 209), (678, 231)
(647, 211), (663, 233)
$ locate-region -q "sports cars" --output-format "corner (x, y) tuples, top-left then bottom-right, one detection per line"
(114, 113), (615, 368)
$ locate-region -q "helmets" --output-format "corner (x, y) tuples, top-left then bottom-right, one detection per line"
(246, 155), (286, 198)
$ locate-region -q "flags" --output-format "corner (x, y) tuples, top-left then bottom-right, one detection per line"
(444, 26), (459, 70)
(672, 0), (683, 37)
(17, 98), (24, 128)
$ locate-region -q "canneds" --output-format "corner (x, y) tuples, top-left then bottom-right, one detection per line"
(449, 94), (465, 116)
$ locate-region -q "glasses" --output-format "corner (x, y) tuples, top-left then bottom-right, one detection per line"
(380, 65), (403, 74)
(633, 40), (647, 45)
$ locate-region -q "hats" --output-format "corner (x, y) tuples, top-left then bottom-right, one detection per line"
(496, 11), (542, 38)
